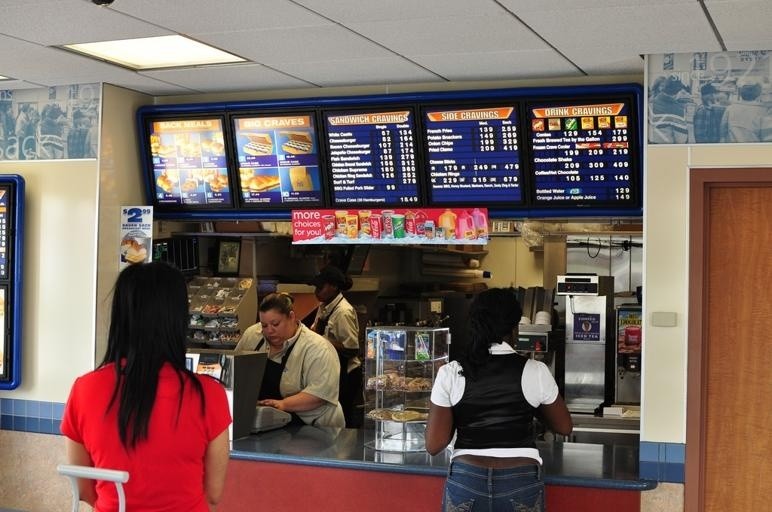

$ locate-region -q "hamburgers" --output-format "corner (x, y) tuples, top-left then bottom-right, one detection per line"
(157, 170), (229, 193)
(150, 134), (224, 159)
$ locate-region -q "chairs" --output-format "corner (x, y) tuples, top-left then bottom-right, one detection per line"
(53, 460), (138, 510)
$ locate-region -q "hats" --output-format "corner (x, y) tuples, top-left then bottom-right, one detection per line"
(307, 265), (346, 286)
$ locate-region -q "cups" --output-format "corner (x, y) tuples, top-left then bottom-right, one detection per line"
(321, 211), (426, 241)
(535, 310), (551, 325)
(624, 325), (642, 345)
(134, 236), (151, 264)
(519, 316), (531, 325)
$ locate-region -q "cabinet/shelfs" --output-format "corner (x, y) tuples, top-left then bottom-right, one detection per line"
(359, 321), (456, 457)
(182, 267), (260, 350)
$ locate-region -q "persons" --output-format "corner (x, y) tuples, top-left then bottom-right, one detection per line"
(2, 92), (99, 161)
(232, 291), (346, 430)
(301, 269), (367, 425)
(423, 279), (574, 511)
(647, 74), (771, 144)
(58, 261), (230, 511)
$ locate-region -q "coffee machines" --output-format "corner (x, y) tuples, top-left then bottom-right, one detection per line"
(614, 305), (642, 408)
(555, 272), (615, 417)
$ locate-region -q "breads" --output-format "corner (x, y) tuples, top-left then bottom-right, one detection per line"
(282, 131), (313, 154)
(239, 168), (280, 193)
(121, 240), (148, 264)
(241, 132), (272, 156)
